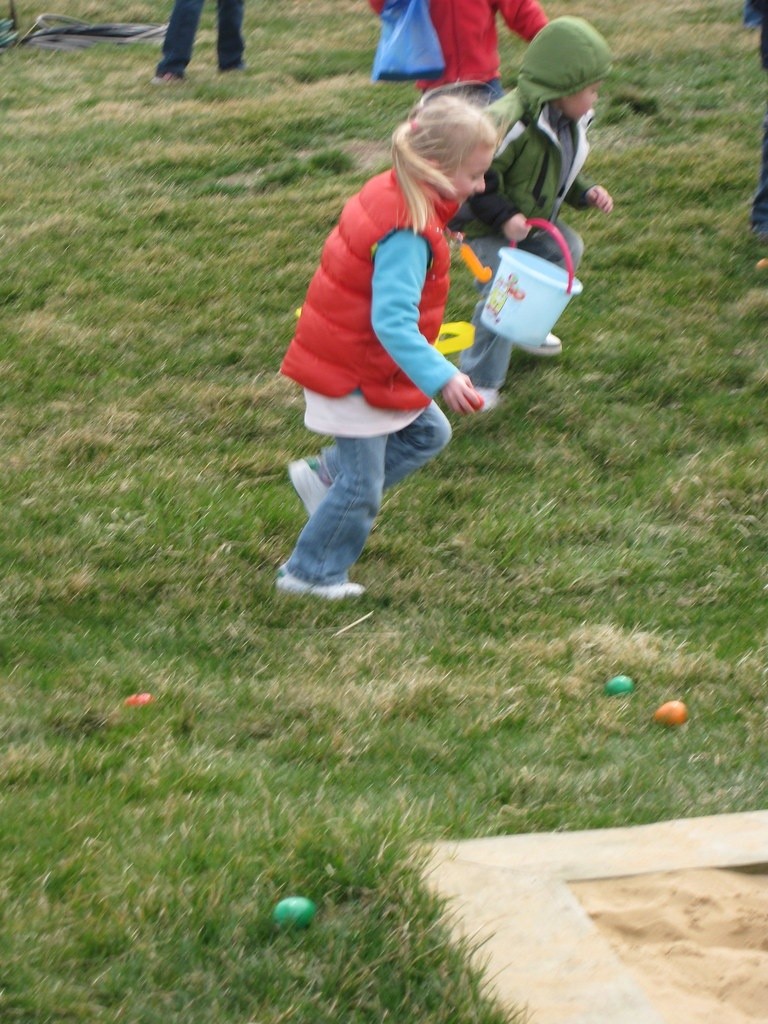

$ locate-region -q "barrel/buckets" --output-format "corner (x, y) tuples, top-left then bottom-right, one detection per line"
(481, 219), (582, 348)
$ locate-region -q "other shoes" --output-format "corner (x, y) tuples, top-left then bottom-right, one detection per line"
(150, 72), (179, 84)
(518, 331), (562, 356)
(476, 388), (495, 411)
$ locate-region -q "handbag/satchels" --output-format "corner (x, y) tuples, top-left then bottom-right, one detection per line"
(370, 0), (446, 82)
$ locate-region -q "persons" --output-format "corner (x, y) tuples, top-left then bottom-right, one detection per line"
(369, 0), (548, 109)
(277, 86), (497, 600)
(151, 0), (246, 91)
(743, 0), (768, 246)
(454, 15), (616, 417)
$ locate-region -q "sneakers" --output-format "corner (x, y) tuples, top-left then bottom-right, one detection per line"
(276, 563), (365, 598)
(288, 453), (335, 516)
